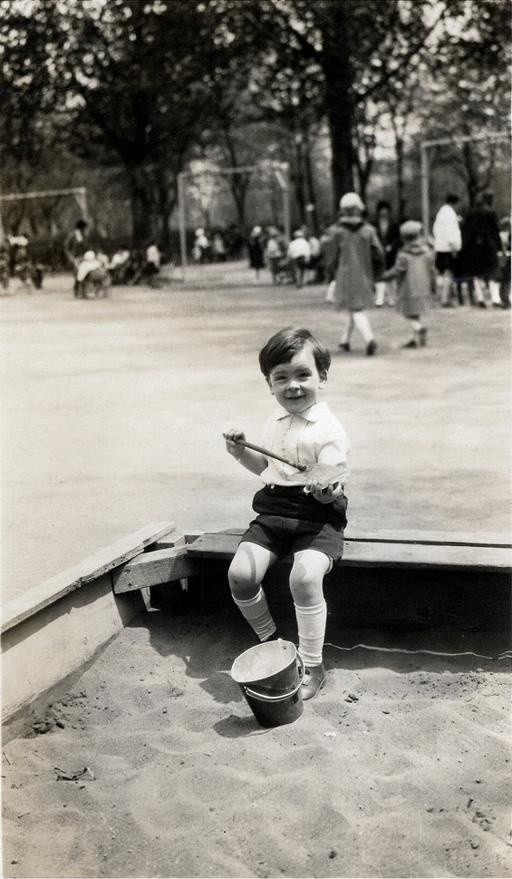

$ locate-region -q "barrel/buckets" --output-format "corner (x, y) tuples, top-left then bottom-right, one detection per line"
(230, 637), (307, 729)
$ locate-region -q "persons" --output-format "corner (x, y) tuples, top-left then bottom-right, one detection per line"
(63, 219), (167, 299)
(370, 192), (512, 309)
(321, 192), (387, 356)
(193, 222), (339, 289)
(2, 227), (44, 289)
(223, 327), (352, 703)
(382, 218), (438, 349)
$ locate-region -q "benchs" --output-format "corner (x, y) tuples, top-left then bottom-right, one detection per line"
(193, 527), (512, 660)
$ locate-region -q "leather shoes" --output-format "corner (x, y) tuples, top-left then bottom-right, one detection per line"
(298, 661), (328, 702)
